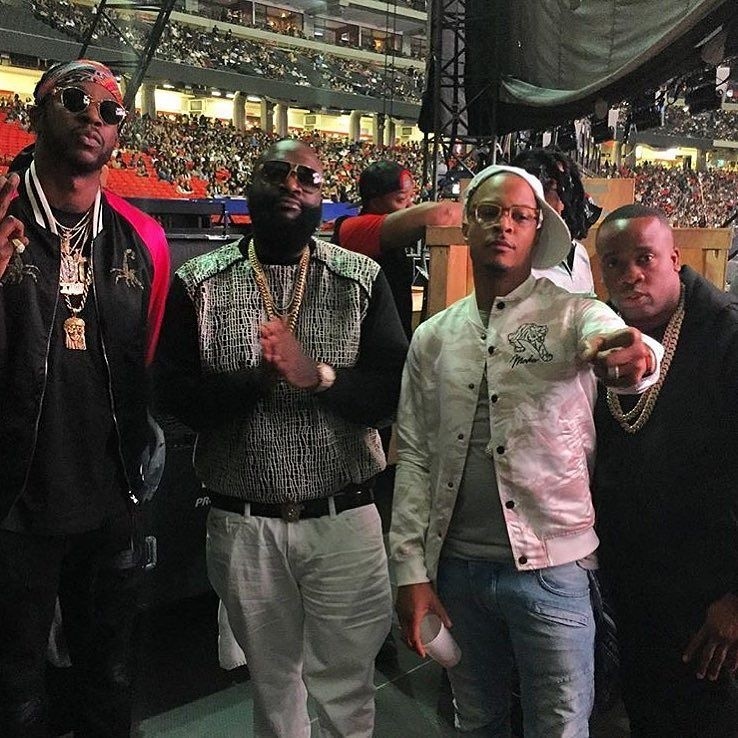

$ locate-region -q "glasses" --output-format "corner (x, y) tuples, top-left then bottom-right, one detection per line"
(251, 160), (324, 194)
(36, 86), (130, 128)
(467, 201), (541, 227)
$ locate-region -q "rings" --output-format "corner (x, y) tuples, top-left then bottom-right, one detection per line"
(607, 366), (620, 383)
(398, 625), (402, 632)
(11, 239), (25, 254)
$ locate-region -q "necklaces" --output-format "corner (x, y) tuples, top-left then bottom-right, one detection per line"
(607, 284), (685, 434)
(53, 211), (96, 351)
(248, 237), (311, 336)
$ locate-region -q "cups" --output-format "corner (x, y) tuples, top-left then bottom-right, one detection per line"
(420, 612), (462, 669)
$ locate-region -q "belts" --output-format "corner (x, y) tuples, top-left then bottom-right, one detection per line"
(207, 486), (376, 524)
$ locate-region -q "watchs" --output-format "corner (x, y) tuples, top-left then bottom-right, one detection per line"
(644, 348), (656, 376)
(314, 362), (336, 394)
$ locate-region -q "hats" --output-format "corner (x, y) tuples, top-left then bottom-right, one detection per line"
(356, 160), (416, 201)
(459, 164), (572, 269)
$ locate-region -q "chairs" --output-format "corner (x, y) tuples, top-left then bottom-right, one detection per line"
(0, 110), (252, 225)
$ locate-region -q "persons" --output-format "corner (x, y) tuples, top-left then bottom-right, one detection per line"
(589, 204), (738, 738)
(330, 160), (464, 465)
(511, 146), (598, 297)
(386, 165), (665, 738)
(0, 59), (172, 738)
(152, 141), (408, 738)
(0, 8), (738, 230)
